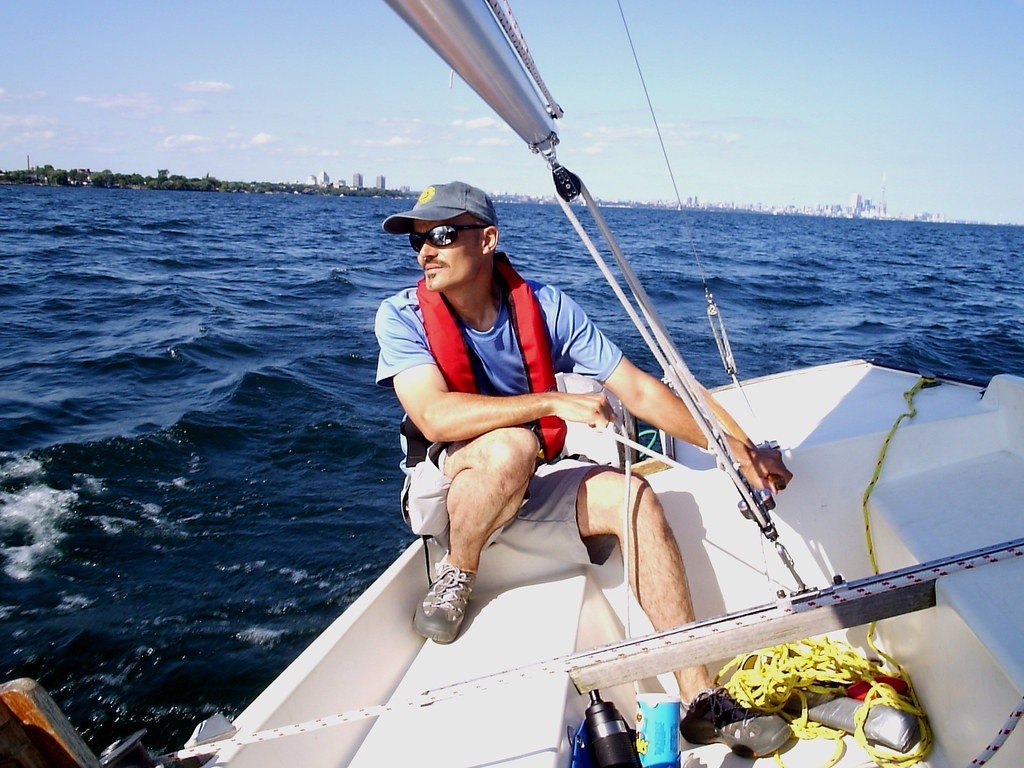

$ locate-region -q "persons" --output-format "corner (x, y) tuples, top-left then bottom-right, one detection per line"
(375, 182), (794, 757)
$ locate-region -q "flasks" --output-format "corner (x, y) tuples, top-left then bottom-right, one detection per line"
(585, 689), (638, 768)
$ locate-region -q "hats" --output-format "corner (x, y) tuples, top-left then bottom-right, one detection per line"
(382, 182), (498, 234)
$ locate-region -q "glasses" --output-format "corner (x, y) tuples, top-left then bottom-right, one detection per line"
(409, 224), (490, 252)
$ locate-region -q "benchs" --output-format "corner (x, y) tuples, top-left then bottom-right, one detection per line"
(340, 566), (668, 768)
(868, 450), (1024, 768)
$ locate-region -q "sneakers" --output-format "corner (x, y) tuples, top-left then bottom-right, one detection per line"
(680, 683), (792, 758)
(412, 563), (476, 644)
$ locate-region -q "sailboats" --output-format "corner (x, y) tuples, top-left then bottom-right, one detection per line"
(163, 1), (1024, 766)
